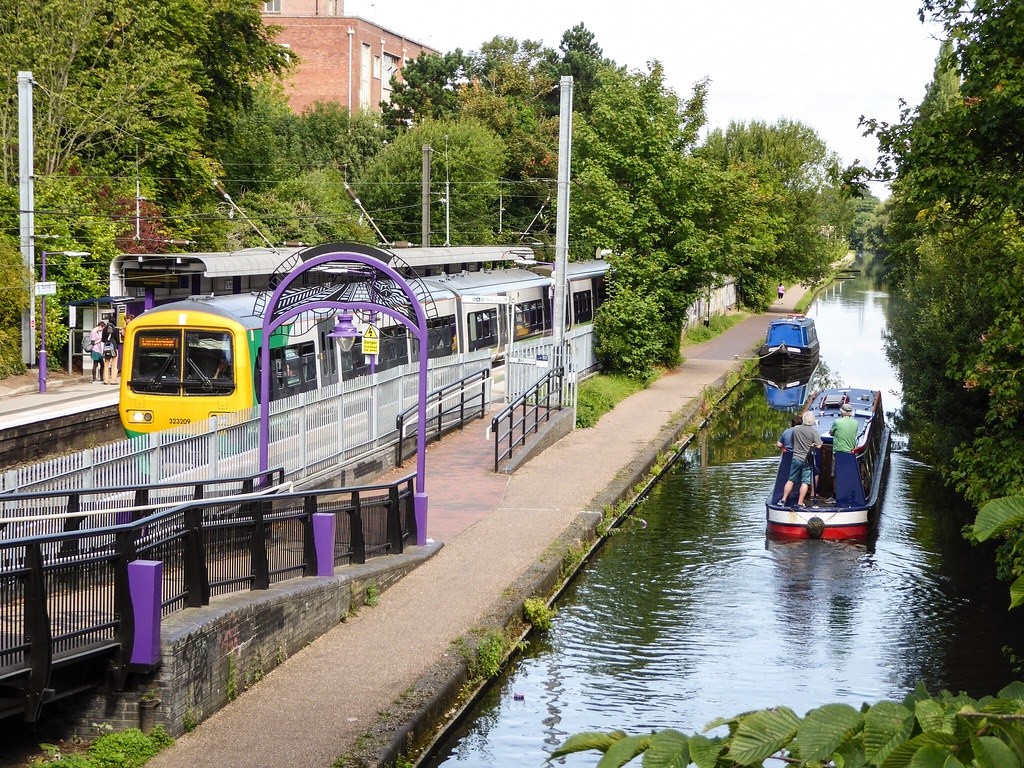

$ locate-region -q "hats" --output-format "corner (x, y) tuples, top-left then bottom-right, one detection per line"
(841, 404), (853, 412)
(803, 410), (816, 426)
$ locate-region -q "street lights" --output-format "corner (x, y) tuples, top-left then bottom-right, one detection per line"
(39, 249), (93, 394)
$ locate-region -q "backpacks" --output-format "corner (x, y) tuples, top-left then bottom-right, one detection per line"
(82, 335), (93, 353)
(103, 342), (117, 359)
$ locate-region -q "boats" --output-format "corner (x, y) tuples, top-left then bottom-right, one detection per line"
(759, 313), (819, 368)
(763, 359), (821, 413)
(766, 387), (892, 541)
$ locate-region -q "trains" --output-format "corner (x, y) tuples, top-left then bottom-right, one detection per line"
(119, 258), (612, 473)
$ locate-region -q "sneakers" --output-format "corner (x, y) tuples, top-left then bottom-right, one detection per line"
(824, 497), (836, 504)
(777, 500), (785, 506)
(794, 503), (808, 511)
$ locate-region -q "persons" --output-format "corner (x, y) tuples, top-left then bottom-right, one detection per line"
(90, 321), (106, 383)
(211, 358), (231, 379)
(829, 404), (865, 507)
(778, 283), (784, 305)
(121, 314), (133, 341)
(777, 411), (823, 508)
(102, 323), (119, 385)
